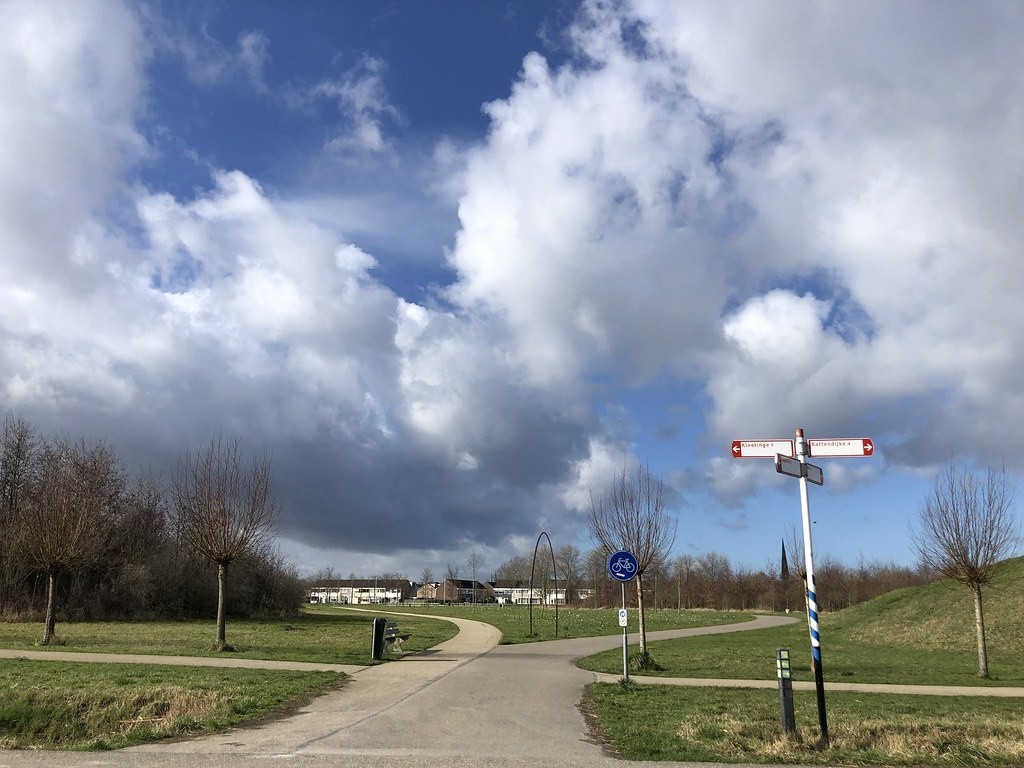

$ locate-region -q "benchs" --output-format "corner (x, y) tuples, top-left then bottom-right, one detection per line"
(372, 622), (412, 654)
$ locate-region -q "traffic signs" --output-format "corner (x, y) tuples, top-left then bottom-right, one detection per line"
(808, 437), (874, 458)
(731, 440), (794, 458)
